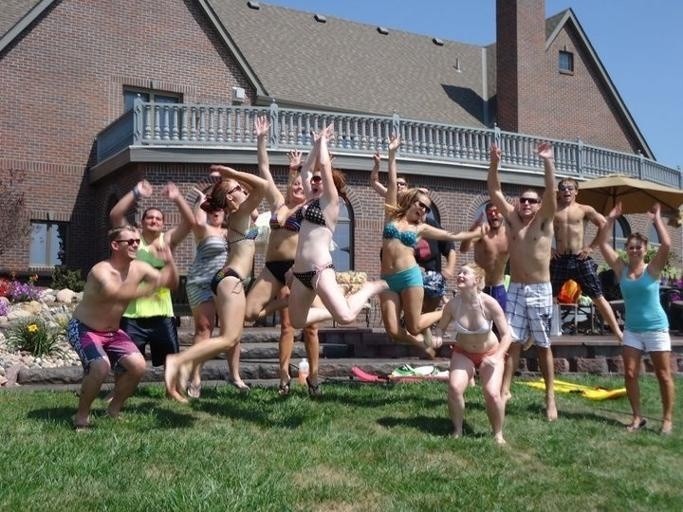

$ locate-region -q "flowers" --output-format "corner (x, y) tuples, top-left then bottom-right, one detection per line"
(0, 273), (42, 316)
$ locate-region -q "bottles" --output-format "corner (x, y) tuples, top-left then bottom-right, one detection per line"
(297, 357), (312, 390)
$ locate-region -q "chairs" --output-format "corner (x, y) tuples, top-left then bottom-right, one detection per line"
(333, 271), (370, 327)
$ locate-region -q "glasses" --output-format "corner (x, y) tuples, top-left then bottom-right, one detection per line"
(518, 197), (542, 205)
(310, 175), (323, 183)
(117, 238), (141, 246)
(415, 199), (431, 214)
(227, 185), (243, 193)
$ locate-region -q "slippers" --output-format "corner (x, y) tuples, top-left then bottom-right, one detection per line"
(278, 377), (291, 396)
(624, 416), (649, 432)
(304, 375), (322, 398)
(185, 382), (203, 400)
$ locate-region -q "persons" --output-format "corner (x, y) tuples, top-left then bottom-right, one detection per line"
(66, 114), (673, 447)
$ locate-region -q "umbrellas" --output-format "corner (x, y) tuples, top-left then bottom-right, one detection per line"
(555, 175), (683, 251)
